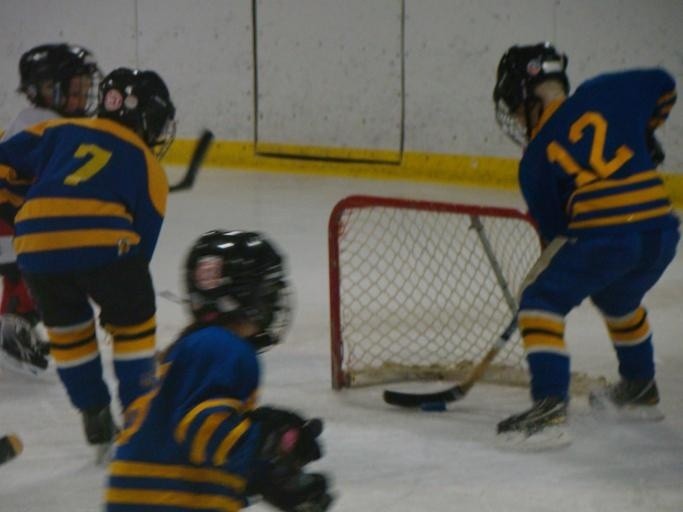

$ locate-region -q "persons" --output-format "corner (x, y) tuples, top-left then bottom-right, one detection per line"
(492, 41), (682, 433)
(104, 229), (333, 512)
(1, 69), (176, 446)
(0, 43), (106, 367)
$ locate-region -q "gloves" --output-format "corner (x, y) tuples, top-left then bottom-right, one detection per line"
(252, 408), (322, 472)
(248, 470), (331, 511)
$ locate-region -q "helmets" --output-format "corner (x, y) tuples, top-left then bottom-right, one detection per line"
(493, 44), (569, 149)
(187, 229), (289, 349)
(20, 44), (102, 117)
(98, 68), (176, 159)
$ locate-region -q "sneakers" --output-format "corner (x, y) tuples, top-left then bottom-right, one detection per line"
(1, 316), (48, 369)
(498, 396), (568, 430)
(590, 379), (658, 409)
(83, 404), (120, 444)
